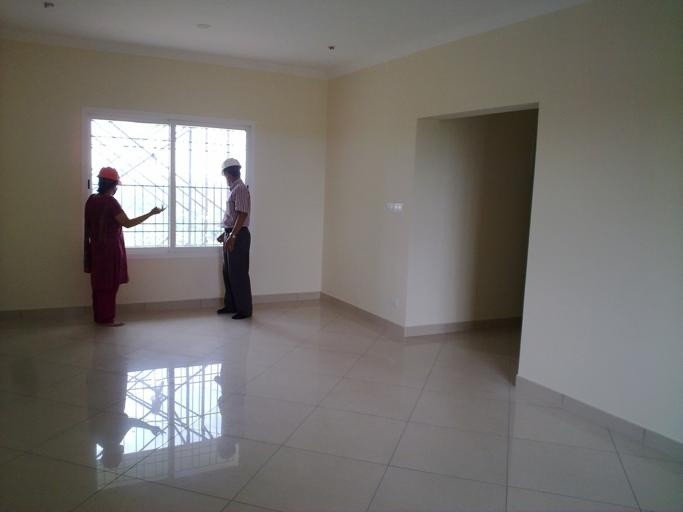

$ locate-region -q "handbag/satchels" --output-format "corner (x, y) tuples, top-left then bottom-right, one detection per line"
(84, 228), (91, 274)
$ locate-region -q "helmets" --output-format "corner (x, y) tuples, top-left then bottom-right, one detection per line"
(96, 167), (121, 183)
(221, 158), (241, 175)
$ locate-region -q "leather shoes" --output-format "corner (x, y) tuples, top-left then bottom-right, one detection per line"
(231, 313), (251, 321)
(215, 306), (233, 315)
(104, 320), (126, 327)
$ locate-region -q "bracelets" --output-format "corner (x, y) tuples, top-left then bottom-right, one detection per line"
(229, 231), (238, 239)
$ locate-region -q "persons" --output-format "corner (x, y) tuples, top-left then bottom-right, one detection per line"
(82, 166), (168, 325)
(215, 158), (257, 320)
(85, 328), (165, 466)
(210, 322), (250, 462)
